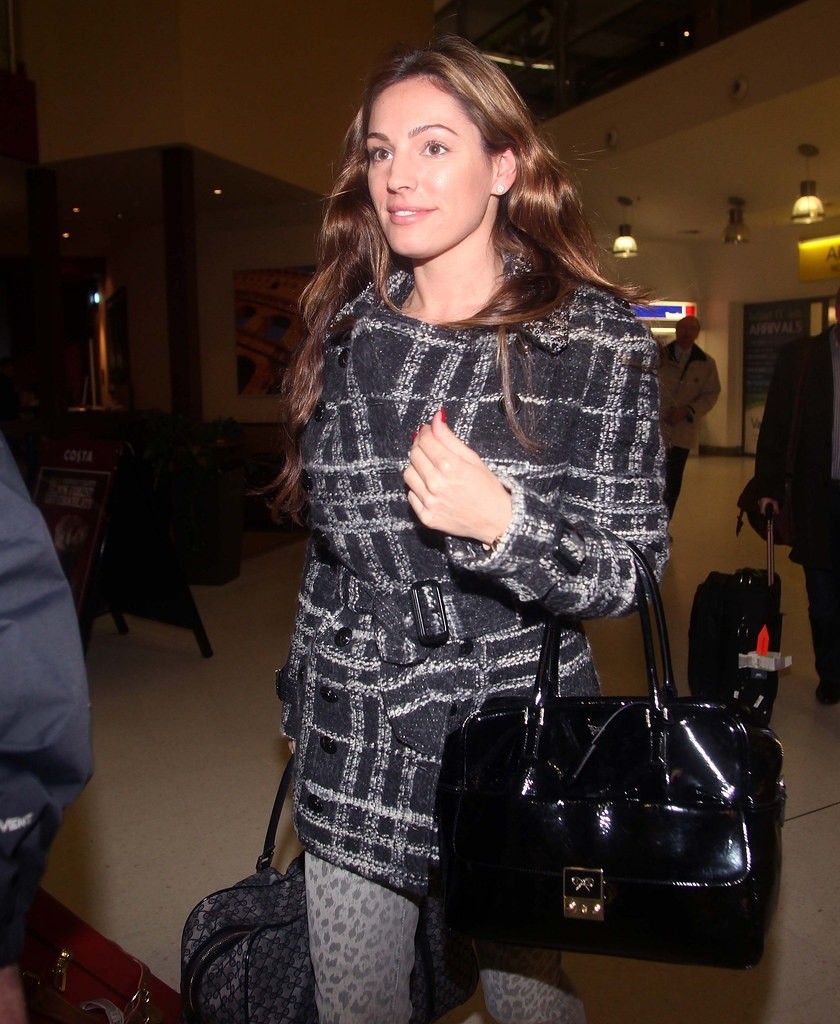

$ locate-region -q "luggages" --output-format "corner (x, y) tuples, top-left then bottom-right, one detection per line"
(687, 502), (784, 727)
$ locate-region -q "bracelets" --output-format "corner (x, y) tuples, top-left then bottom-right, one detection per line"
(482, 534), (504, 558)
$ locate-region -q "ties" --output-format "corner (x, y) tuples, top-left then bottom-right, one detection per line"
(678, 351), (687, 373)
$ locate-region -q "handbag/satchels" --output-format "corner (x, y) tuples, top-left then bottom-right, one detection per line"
(180, 751), (480, 1024)
(18, 883), (181, 1024)
(735, 476), (800, 547)
(430, 539), (790, 972)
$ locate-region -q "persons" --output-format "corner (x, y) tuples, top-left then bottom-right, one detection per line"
(737, 291), (840, 705)
(0, 436), (94, 1024)
(261, 36), (668, 1024)
(659, 317), (720, 544)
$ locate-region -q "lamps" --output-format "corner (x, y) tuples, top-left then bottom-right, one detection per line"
(722, 194), (753, 245)
(612, 196), (638, 258)
(790, 144), (826, 223)
